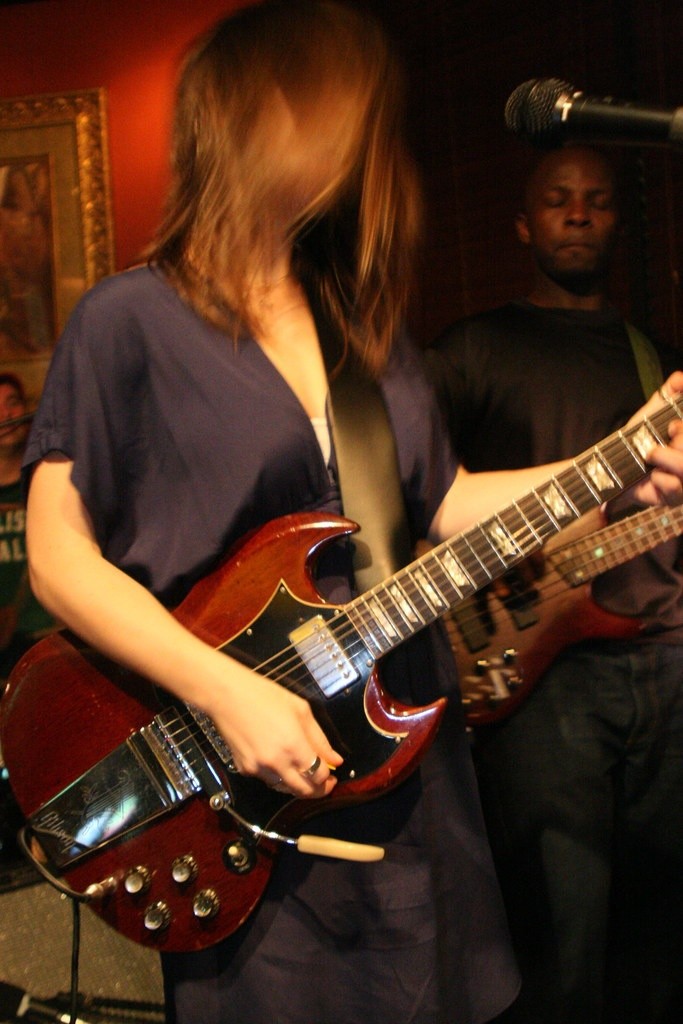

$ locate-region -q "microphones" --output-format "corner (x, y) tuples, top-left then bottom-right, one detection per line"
(504, 77), (683, 153)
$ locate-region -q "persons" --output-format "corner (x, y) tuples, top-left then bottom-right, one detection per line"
(23, 6), (683, 1023)
(422, 140), (683, 1019)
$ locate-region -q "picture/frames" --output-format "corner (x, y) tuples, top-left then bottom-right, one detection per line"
(0, 87), (119, 420)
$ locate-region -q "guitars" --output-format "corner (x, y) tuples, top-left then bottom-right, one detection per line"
(416, 496), (683, 725)
(0, 398), (683, 954)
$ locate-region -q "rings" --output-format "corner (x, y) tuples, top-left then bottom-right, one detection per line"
(302, 757), (321, 776)
(267, 778), (284, 791)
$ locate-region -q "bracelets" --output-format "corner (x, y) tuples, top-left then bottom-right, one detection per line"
(597, 498), (615, 528)
